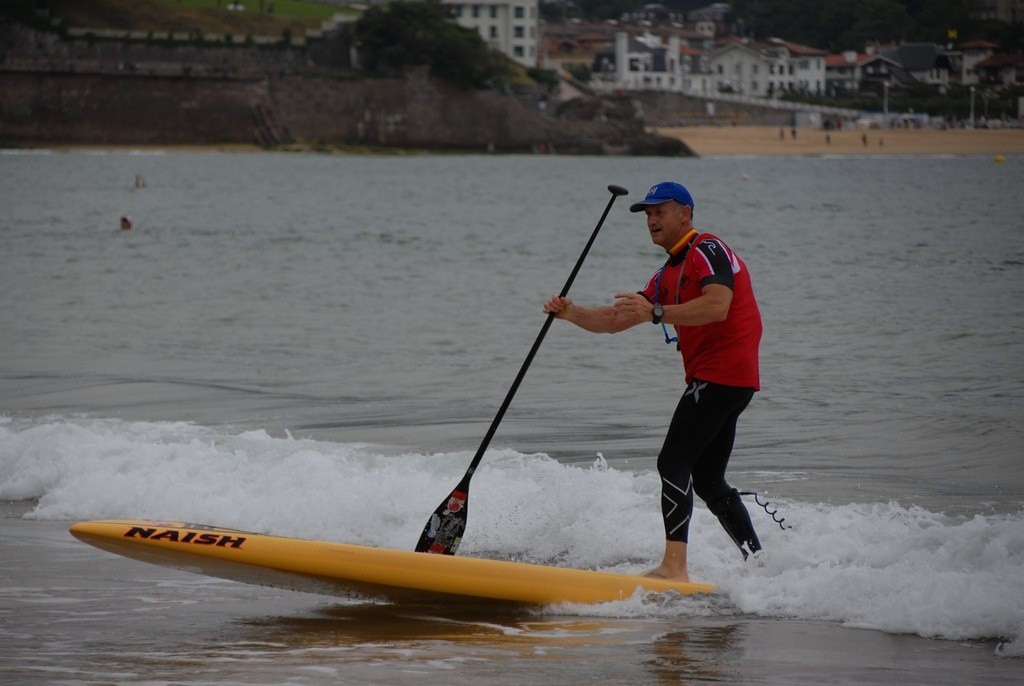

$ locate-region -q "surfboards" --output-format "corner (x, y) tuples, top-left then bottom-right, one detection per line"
(69, 518), (719, 607)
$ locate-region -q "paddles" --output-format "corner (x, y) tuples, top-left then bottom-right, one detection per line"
(414, 185), (631, 557)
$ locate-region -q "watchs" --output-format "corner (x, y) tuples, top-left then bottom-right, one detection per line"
(652, 303), (664, 324)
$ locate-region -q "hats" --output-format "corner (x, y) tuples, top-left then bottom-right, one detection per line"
(630, 182), (694, 213)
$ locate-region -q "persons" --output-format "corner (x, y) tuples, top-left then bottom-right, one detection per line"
(543, 182), (762, 581)
(120, 218), (131, 229)
(791, 128), (797, 138)
(862, 134), (867, 145)
(780, 128), (785, 139)
(823, 115), (830, 127)
(826, 134), (831, 144)
(835, 114), (841, 129)
